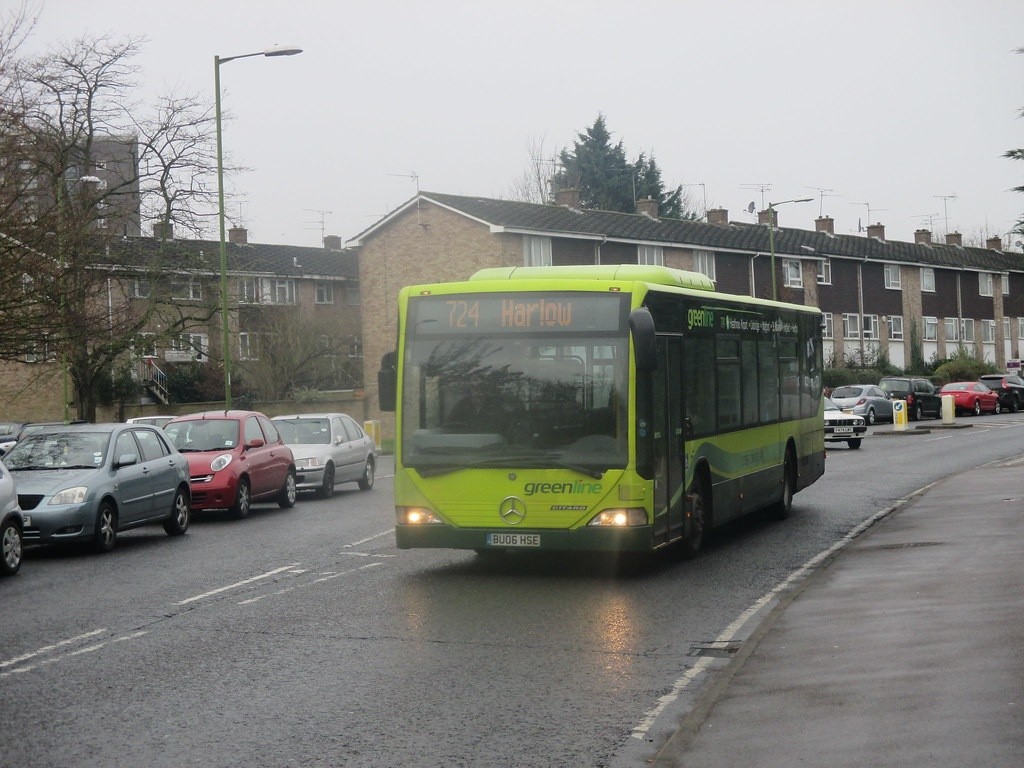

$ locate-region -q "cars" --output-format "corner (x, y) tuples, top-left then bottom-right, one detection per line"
(0, 423), (66, 580)
(939, 381), (1000, 416)
(122, 416), (193, 442)
(160, 410), (297, 518)
(1, 422), (193, 553)
(824, 396), (868, 449)
(270, 411), (377, 497)
(829, 383), (895, 424)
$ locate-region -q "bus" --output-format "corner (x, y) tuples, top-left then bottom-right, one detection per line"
(375, 264), (826, 559)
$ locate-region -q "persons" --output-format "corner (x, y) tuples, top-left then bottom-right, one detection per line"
(442, 374), (509, 437)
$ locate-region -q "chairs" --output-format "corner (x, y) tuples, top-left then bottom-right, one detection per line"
(184, 427), (206, 450)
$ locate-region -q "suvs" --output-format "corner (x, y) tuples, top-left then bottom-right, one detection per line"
(976, 373), (1024, 413)
(878, 375), (942, 421)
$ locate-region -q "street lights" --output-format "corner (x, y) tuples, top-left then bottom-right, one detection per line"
(57, 175), (101, 422)
(212, 46), (304, 411)
(768, 197), (814, 301)
